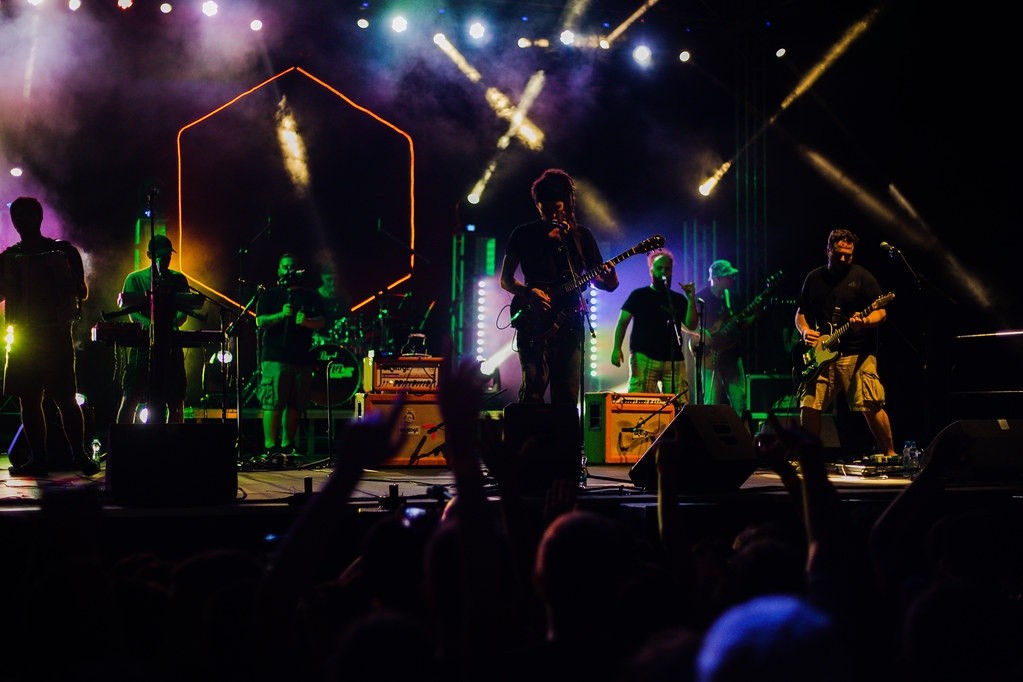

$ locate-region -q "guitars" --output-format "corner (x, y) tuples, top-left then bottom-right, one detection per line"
(792, 290), (895, 384)
(509, 229), (666, 340)
(688, 270), (783, 369)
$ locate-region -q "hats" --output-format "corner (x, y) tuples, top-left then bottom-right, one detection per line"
(709, 260), (738, 278)
(149, 234), (177, 255)
(695, 593), (831, 682)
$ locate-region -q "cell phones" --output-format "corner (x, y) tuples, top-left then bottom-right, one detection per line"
(398, 504), (430, 531)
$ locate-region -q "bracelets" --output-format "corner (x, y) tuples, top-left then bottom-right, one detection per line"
(688, 304), (697, 311)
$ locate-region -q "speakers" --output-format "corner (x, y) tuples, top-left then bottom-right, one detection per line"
(104, 422), (238, 501)
(919, 420), (1023, 488)
(628, 403), (765, 496)
(757, 414), (842, 462)
(7, 395), (97, 472)
(353, 393), (447, 467)
(503, 402), (581, 495)
(584, 393), (678, 466)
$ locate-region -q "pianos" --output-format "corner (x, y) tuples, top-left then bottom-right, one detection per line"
(91, 292), (226, 425)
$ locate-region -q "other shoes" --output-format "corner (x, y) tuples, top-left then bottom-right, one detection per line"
(265, 446), (283, 456)
(279, 444), (304, 455)
(81, 460), (100, 475)
(9, 462), (48, 477)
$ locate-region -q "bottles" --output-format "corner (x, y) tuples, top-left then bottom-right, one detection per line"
(580, 446), (588, 486)
(907, 442), (919, 468)
(902, 441), (910, 477)
(91, 435), (102, 466)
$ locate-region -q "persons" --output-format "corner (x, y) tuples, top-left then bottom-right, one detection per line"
(501, 167), (619, 482)
(254, 251), (327, 459)
(0, 197), (101, 476)
(612, 247), (772, 421)
(0, 390), (1023, 682)
(789, 229), (899, 472)
(116, 234), (190, 425)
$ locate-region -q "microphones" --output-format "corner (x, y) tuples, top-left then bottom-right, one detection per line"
(427, 423), (444, 434)
(621, 427), (641, 432)
(696, 297), (706, 304)
(550, 219), (569, 230)
(147, 184), (160, 206)
(661, 275), (669, 293)
(879, 241), (901, 254)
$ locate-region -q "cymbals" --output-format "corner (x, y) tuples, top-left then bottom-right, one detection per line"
(374, 316), (399, 318)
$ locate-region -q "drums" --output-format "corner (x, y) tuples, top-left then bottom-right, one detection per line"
(307, 342), (361, 408)
